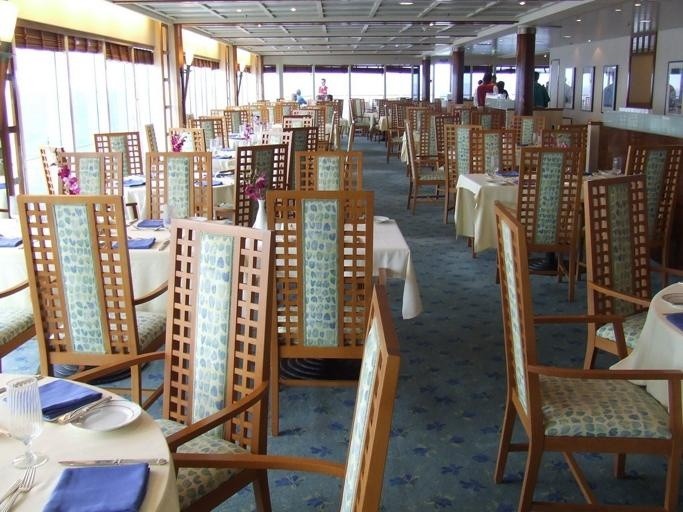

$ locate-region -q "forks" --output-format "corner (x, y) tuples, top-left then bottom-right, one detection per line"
(1, 464), (36, 512)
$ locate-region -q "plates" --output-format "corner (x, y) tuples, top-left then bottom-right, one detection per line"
(189, 217), (207, 221)
(67, 399), (142, 430)
(661, 292), (682, 309)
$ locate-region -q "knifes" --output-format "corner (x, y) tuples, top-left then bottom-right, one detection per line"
(57, 458), (166, 465)
(63, 395), (111, 425)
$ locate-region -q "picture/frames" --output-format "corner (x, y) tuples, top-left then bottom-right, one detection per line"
(549, 59), (618, 114)
(663, 60), (682, 117)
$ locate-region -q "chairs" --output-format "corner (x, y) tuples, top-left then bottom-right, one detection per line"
(66, 219), (275, 510)
(492, 200), (682, 512)
(1, 97), (682, 384)
(17, 194), (170, 411)
(173, 282), (400, 511)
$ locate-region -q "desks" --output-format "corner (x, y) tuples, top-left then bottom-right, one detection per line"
(2, 372), (179, 510)
(609, 282), (683, 412)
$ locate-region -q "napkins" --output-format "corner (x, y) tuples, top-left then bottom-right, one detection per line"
(45, 463), (150, 510)
(38, 379), (102, 419)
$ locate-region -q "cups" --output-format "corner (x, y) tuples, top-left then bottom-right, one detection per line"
(486, 156), (498, 179)
(209, 140), (217, 154)
(612, 157), (621, 174)
(126, 203), (138, 220)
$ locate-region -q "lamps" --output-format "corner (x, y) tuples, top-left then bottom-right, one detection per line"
(181, 42), (195, 105)
(0, 0), (18, 74)
(237, 51), (248, 97)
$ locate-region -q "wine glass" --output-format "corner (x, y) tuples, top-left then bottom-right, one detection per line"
(5, 376), (48, 468)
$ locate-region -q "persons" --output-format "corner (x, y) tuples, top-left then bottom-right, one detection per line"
(473, 70), (509, 108)
(604, 71), (614, 107)
(292, 77), (332, 104)
(564, 78), (570, 103)
(534, 71), (550, 107)
